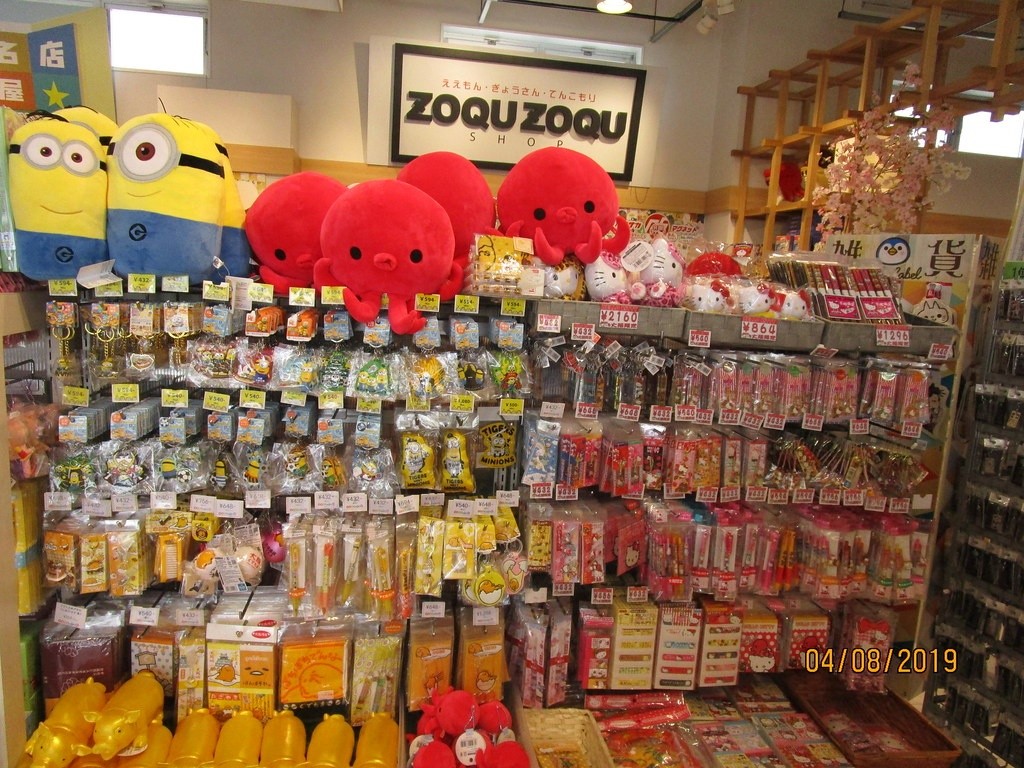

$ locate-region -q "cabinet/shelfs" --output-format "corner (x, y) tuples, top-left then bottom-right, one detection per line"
(731, 0), (1024, 251)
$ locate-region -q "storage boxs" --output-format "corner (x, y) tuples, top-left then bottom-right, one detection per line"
(526, 298), (686, 338)
(682, 307), (826, 352)
(814, 315), (954, 354)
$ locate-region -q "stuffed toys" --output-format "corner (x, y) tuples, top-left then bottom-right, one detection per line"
(184, 118), (259, 279)
(243, 172), (349, 295)
(395, 153), (500, 273)
(52, 103), (112, 155)
(316, 181), (464, 332)
(106, 112), (224, 287)
(7, 111), (111, 282)
(496, 148), (630, 268)
(405, 686), (532, 768)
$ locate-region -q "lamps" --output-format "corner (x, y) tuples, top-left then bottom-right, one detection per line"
(696, 14), (718, 34)
(717, 0), (735, 16)
(595, 0), (633, 14)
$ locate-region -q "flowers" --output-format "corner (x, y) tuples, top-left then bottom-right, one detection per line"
(811, 60), (971, 251)
